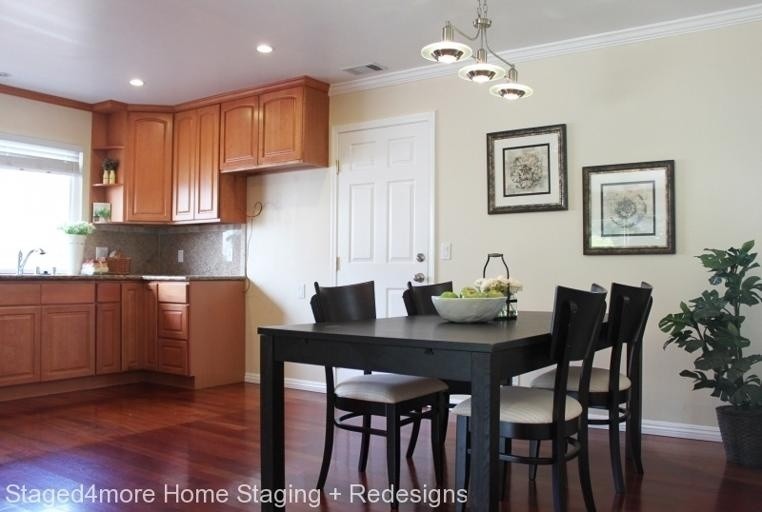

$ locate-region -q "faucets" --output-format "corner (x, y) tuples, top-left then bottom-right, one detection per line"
(18, 248), (45, 275)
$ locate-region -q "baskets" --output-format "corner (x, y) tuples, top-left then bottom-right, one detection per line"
(107, 249), (132, 274)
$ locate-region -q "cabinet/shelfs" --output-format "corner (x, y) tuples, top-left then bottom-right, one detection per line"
(126, 104), (175, 227)
(96, 280), (122, 390)
(172, 94), (247, 227)
(123, 281), (244, 390)
(0, 281), (96, 405)
(90, 99), (127, 226)
(219, 75), (331, 177)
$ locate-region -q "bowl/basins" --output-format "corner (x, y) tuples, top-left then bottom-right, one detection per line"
(431, 295), (505, 322)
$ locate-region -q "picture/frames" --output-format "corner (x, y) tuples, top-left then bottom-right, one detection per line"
(485, 123), (569, 216)
(581, 159), (678, 258)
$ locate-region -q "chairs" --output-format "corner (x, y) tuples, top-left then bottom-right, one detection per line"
(526, 279), (656, 494)
(401, 279), (514, 503)
(451, 283), (608, 511)
(308, 280), (450, 512)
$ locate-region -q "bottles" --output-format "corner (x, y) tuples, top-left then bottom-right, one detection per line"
(102, 169), (116, 184)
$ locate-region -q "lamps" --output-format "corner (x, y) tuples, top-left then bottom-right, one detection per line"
(419, 1), (536, 102)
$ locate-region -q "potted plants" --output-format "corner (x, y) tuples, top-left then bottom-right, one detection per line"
(658, 239), (762, 470)
(102, 157), (110, 184)
(110, 159), (119, 183)
(65, 219), (97, 276)
